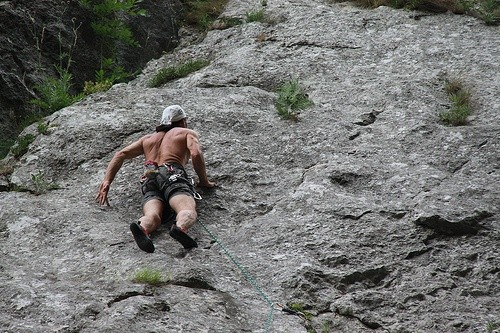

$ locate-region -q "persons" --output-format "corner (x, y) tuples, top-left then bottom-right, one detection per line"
(94, 105), (217, 253)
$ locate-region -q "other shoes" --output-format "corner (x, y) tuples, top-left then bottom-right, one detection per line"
(170, 224), (198, 248)
(130, 221), (155, 253)
